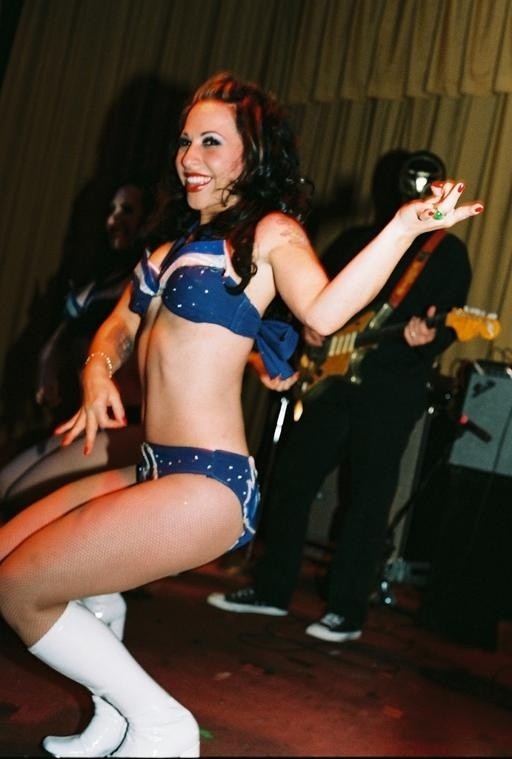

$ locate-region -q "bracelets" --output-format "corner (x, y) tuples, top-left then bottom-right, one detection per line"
(85, 352), (112, 380)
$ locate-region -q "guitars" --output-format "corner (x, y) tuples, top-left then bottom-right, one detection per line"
(290, 303), (501, 401)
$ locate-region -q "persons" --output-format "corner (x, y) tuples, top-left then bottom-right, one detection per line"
(2, 71), (484, 759)
(207, 151), (472, 643)
(35, 176), (182, 436)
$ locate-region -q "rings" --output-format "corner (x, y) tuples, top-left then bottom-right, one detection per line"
(432, 205), (445, 221)
(411, 331), (416, 339)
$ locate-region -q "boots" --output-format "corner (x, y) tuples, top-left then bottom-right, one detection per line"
(27, 593), (200, 757)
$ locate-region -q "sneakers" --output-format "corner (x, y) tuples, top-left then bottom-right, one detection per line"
(206, 585), (289, 616)
(306, 610), (363, 643)
